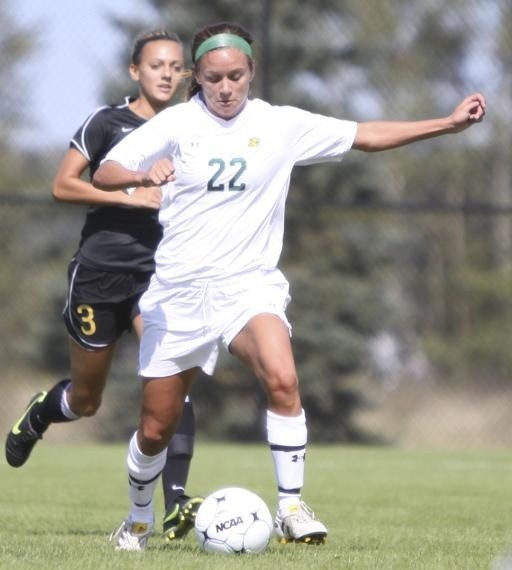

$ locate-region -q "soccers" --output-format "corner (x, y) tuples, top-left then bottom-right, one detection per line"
(195, 487), (272, 556)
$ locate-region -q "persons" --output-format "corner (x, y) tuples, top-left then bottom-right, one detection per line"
(91, 22), (487, 557)
(5, 25), (207, 545)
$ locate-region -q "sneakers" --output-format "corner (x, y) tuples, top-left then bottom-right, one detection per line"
(6, 391), (51, 467)
(162, 497), (204, 541)
(275, 501), (327, 544)
(111, 515), (154, 553)
